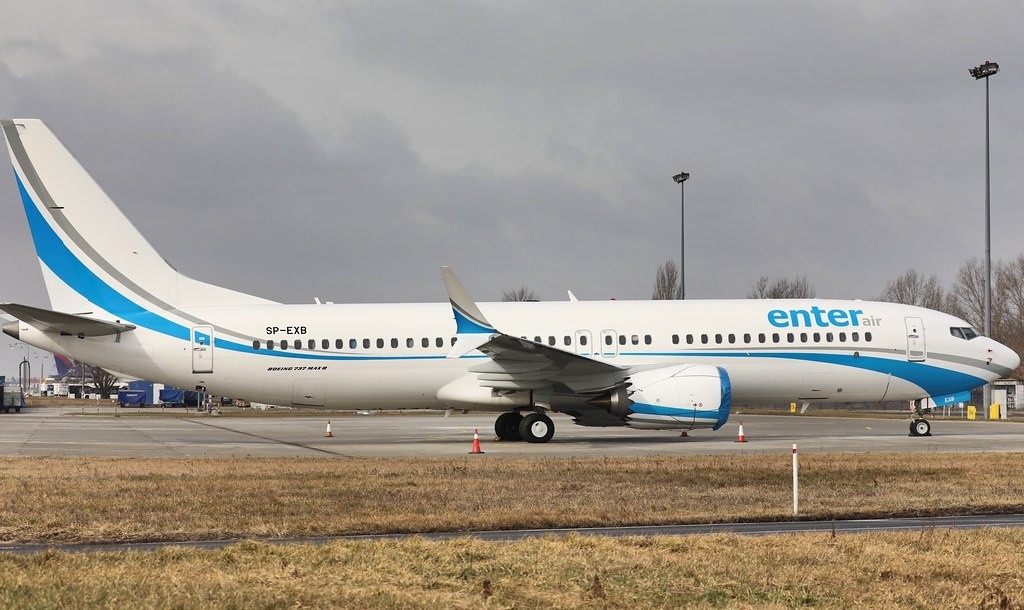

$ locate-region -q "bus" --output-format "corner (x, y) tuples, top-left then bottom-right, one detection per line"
(67, 383), (128, 398)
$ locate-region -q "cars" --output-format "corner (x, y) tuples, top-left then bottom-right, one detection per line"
(354, 410), (370, 416)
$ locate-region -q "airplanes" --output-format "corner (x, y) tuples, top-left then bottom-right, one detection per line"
(0, 118), (1021, 442)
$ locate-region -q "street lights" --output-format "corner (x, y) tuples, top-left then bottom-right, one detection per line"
(13, 342), (30, 362)
(671, 171), (690, 298)
(966, 59), (1000, 418)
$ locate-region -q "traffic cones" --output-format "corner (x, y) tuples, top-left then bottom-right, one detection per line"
(324, 419), (335, 437)
(467, 429), (486, 453)
(733, 419), (749, 443)
